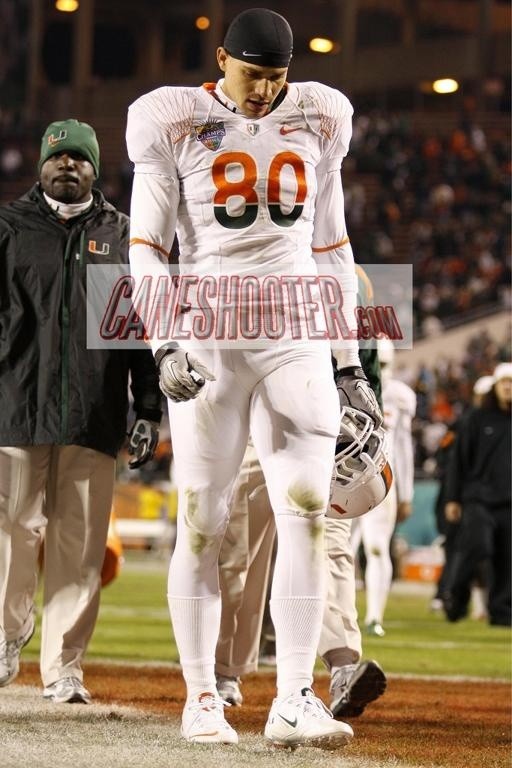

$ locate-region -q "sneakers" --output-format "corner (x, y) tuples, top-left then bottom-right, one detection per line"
(216, 674), (244, 707)
(0, 620), (36, 687)
(42, 677), (92, 705)
(180, 690), (239, 745)
(264, 686), (355, 752)
(327, 657), (389, 719)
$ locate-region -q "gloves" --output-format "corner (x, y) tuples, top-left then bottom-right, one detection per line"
(126, 408), (164, 469)
(335, 366), (385, 432)
(154, 341), (217, 404)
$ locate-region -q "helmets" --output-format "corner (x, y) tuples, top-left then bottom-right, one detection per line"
(323, 405), (393, 520)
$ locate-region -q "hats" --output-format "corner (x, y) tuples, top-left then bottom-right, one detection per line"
(223, 8), (294, 68)
(37, 118), (100, 179)
(377, 332), (512, 394)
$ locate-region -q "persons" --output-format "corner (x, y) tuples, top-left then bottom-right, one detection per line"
(430, 377), (490, 608)
(211, 250), (386, 724)
(441, 364), (510, 622)
(114, 440), (178, 528)
(350, 82), (510, 472)
(363, 324), (417, 638)
(125, 10), (352, 749)
(3, 117), (162, 704)
